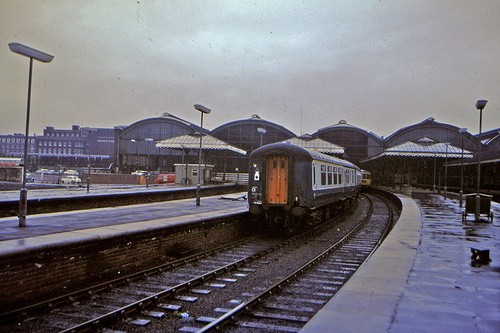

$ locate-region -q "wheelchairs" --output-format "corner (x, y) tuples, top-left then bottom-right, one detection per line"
(462, 193), (493, 223)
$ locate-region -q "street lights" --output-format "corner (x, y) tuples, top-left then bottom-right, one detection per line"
(474, 99), (489, 222)
(193, 104), (213, 205)
(444, 142), (451, 199)
(458, 128), (467, 207)
(9, 41), (54, 226)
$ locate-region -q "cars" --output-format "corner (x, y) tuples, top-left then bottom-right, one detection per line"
(36, 167), (80, 176)
(62, 176), (81, 184)
(25, 174), (34, 182)
(131, 170), (150, 176)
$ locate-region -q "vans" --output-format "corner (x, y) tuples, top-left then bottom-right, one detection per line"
(155, 174), (175, 183)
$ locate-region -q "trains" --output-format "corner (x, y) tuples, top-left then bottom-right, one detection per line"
(246, 141), (363, 230)
(361, 170), (372, 189)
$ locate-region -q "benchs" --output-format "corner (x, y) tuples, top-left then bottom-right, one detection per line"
(467, 243), (491, 265)
